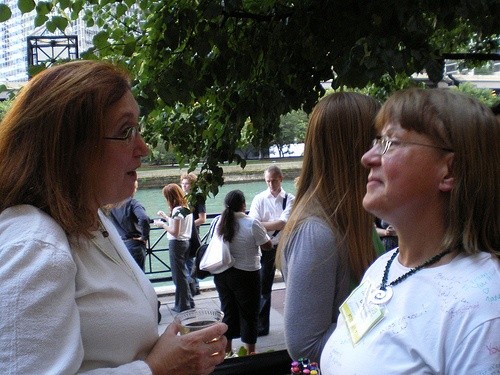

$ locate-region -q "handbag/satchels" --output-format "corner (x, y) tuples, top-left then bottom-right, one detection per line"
(191, 216), (219, 280)
(199, 217), (235, 275)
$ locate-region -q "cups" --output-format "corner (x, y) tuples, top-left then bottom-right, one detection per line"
(154, 219), (161, 225)
(173, 309), (225, 356)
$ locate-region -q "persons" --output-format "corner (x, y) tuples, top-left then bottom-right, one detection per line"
(153, 183), (195, 312)
(247, 165), (298, 338)
(105, 177), (152, 273)
(314, 85), (500, 375)
(279, 94), (385, 366)
(180, 173), (206, 254)
(0, 58), (228, 375)
(209, 189), (272, 359)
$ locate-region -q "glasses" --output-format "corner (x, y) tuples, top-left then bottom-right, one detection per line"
(101, 116), (147, 143)
(372, 135), (456, 154)
